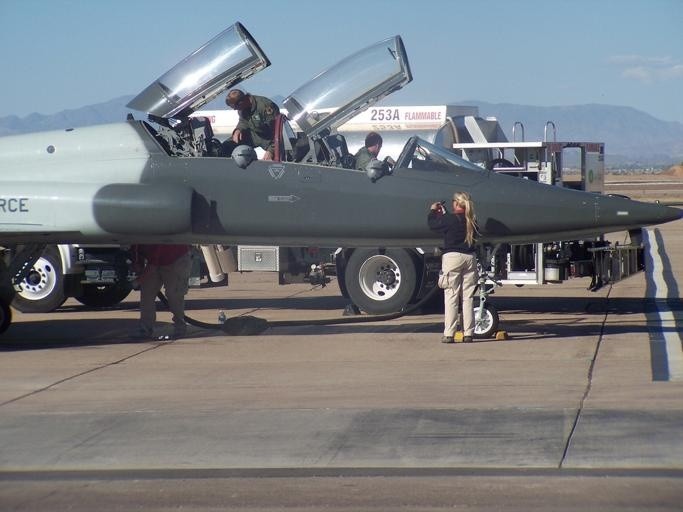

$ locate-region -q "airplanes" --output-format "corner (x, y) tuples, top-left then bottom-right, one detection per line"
(0, 19), (682, 337)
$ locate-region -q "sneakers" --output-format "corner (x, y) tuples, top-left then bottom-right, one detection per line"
(441, 336), (454, 343)
(463, 337), (472, 343)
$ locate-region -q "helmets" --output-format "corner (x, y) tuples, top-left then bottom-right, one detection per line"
(366, 159), (389, 180)
(231, 145), (257, 168)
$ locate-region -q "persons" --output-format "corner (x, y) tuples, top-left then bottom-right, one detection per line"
(427, 191), (484, 343)
(221, 88), (283, 162)
(126, 242), (191, 342)
(354, 130), (393, 175)
